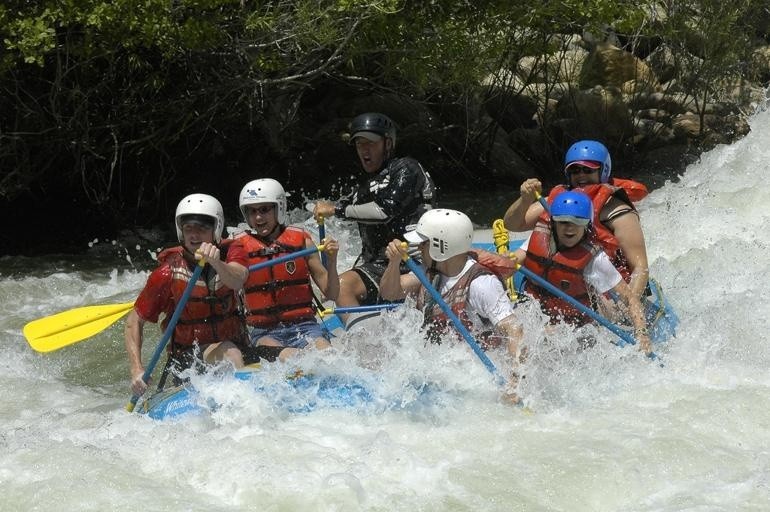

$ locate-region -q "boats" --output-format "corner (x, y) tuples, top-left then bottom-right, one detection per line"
(140, 238), (681, 451)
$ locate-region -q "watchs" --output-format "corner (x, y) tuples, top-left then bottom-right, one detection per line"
(634, 327), (648, 335)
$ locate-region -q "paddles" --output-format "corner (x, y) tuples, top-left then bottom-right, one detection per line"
(23, 239), (334, 352)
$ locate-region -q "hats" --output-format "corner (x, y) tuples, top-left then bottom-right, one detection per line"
(349, 132), (384, 143)
(552, 215), (590, 226)
(565, 160), (601, 173)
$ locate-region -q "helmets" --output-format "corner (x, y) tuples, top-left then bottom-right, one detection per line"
(175, 193), (224, 245)
(350, 114), (395, 151)
(416, 208), (473, 262)
(238, 178), (287, 225)
(565, 140), (612, 184)
(403, 230), (429, 243)
(551, 192), (594, 229)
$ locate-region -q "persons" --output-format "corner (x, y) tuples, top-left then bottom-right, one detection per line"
(313, 112), (437, 326)
(379, 208), (523, 405)
(126, 193), (299, 400)
(500, 191), (653, 354)
(232, 178), (339, 363)
(503, 140), (649, 299)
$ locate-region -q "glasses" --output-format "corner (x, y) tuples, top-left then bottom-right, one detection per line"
(245, 205), (275, 214)
(570, 166), (596, 174)
